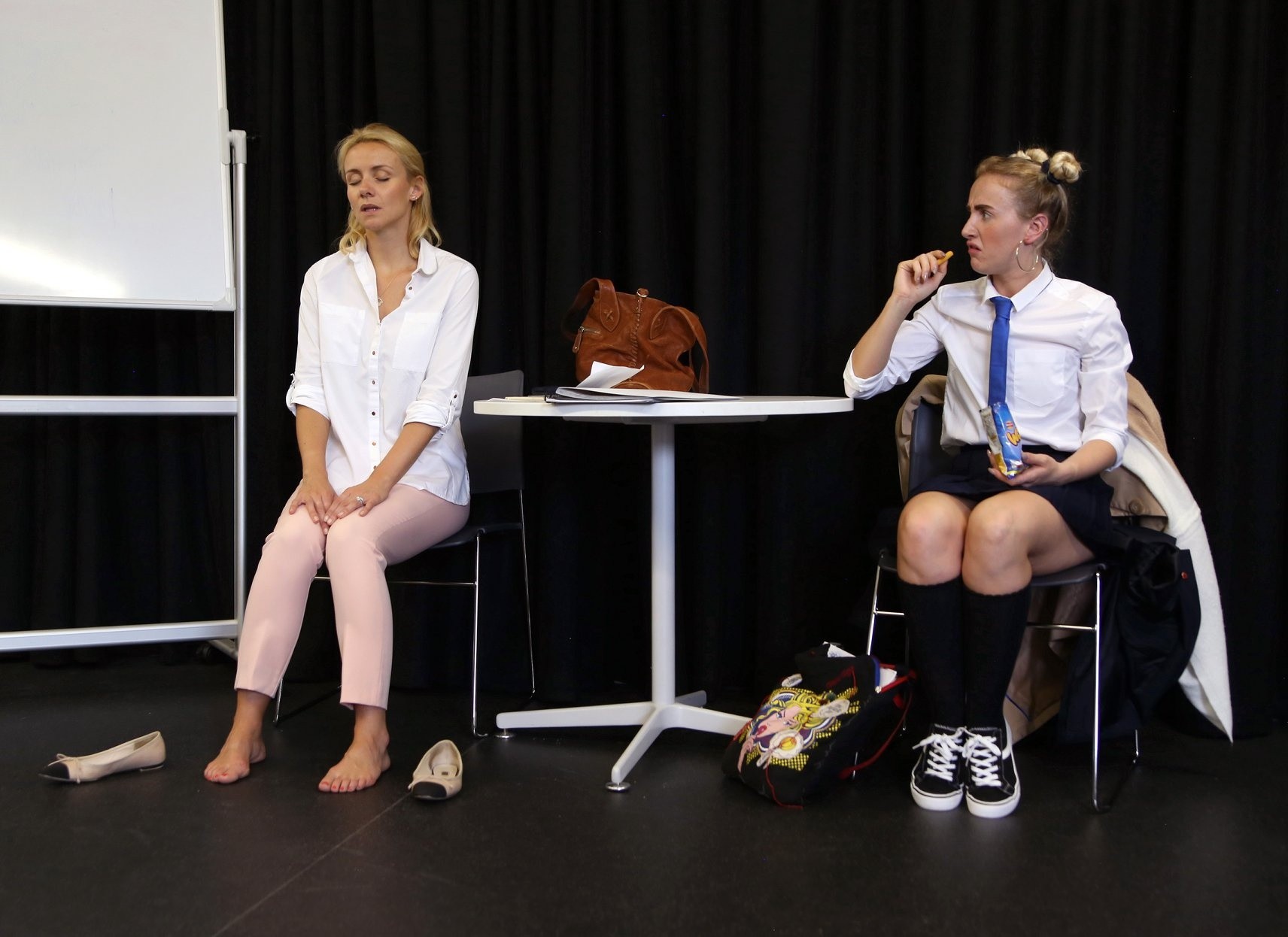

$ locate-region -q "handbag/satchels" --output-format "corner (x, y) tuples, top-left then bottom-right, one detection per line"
(571, 280), (711, 395)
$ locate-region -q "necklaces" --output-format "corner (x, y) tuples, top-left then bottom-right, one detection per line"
(378, 269), (401, 306)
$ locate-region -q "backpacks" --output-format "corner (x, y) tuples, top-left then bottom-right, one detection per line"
(720, 641), (917, 812)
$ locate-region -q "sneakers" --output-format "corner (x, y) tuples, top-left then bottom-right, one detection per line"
(959, 713), (1021, 818)
(911, 719), (967, 811)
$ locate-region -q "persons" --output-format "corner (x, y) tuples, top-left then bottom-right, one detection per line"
(842, 149), (1134, 820)
(204, 122), (479, 796)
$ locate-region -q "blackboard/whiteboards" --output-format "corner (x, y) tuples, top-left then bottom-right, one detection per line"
(0, 0), (236, 312)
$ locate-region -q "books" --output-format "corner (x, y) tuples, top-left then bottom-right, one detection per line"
(544, 387), (744, 403)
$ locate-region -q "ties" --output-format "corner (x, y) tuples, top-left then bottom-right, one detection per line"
(988, 297), (1014, 407)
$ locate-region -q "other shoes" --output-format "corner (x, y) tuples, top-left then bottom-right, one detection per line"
(406, 738), (463, 802)
(37, 731), (167, 782)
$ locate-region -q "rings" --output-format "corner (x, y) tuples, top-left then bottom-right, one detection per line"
(356, 495), (364, 505)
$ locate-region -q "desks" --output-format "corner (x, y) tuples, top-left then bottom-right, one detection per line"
(473, 390), (856, 792)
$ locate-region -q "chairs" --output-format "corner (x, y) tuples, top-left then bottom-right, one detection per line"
(863, 371), (1161, 818)
(273, 370), (538, 737)
